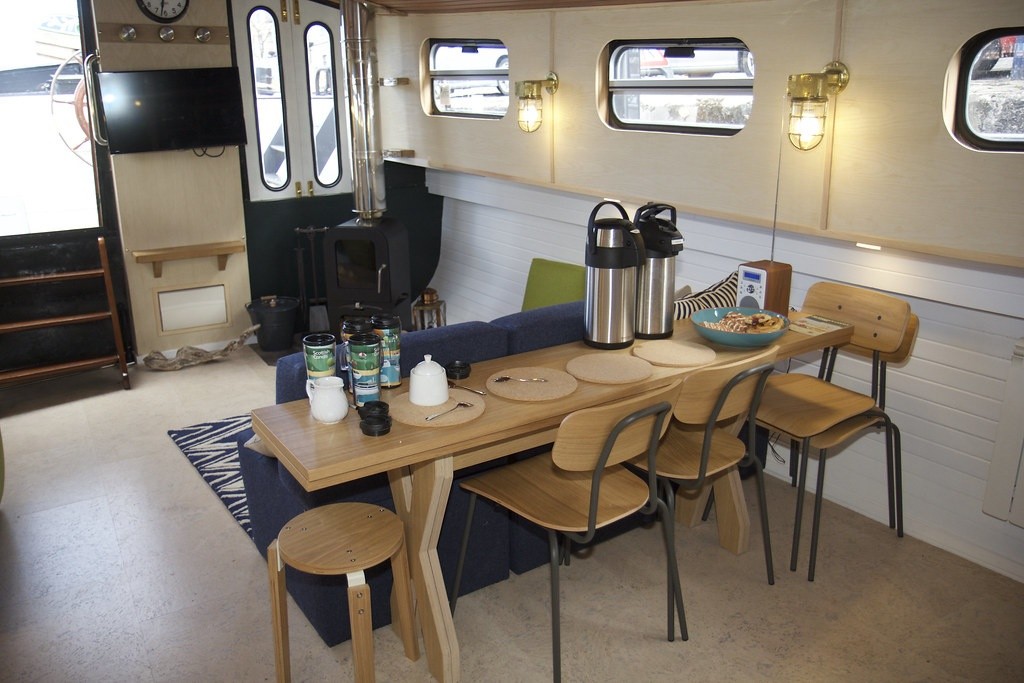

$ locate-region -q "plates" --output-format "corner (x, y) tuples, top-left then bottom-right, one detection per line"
(689, 307), (792, 347)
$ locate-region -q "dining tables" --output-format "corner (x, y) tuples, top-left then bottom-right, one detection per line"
(248, 301), (856, 683)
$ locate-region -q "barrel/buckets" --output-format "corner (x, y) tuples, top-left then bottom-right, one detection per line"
(244, 296), (301, 352)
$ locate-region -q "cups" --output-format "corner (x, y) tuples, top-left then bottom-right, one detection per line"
(408, 355), (449, 406)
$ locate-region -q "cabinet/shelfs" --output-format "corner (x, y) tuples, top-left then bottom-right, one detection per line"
(0, 237), (132, 389)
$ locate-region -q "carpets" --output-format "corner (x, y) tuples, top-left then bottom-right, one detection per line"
(167, 413), (253, 542)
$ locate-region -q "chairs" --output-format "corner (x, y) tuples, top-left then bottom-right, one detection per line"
(698, 280), (911, 573)
(564, 345), (781, 645)
(745, 312), (920, 584)
(448, 376), (692, 683)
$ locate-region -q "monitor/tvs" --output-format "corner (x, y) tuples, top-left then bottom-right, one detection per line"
(96, 66), (247, 155)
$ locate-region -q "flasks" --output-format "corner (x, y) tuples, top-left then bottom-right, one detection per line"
(581, 201), (646, 350)
(632, 203), (687, 339)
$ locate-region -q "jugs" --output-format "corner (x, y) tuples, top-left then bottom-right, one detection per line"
(305, 376), (349, 425)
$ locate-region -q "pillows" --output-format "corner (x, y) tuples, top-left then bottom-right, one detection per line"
(520, 258), (588, 313)
(674, 270), (738, 322)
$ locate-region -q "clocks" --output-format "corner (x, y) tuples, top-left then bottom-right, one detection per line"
(136, 0), (189, 25)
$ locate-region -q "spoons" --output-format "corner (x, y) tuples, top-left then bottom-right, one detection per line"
(494, 375), (544, 382)
(448, 380), (486, 395)
(425, 402), (473, 421)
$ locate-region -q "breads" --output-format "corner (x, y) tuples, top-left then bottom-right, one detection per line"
(698, 311), (784, 333)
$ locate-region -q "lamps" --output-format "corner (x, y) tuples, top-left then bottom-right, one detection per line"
(786, 60), (851, 152)
(517, 70), (559, 133)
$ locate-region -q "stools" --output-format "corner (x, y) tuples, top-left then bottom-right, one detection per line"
(268, 501), (420, 683)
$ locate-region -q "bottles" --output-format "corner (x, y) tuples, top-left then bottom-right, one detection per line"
(412, 288), (447, 331)
(303, 311), (401, 411)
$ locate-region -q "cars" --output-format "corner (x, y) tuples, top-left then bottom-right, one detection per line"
(640, 50), (753, 78)
(434, 46), (509, 99)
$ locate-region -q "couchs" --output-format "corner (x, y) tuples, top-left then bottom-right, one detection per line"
(236, 302), (588, 647)
